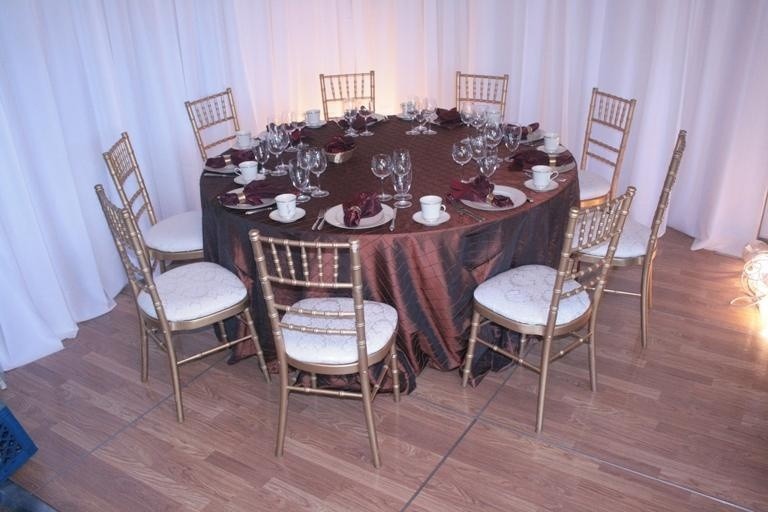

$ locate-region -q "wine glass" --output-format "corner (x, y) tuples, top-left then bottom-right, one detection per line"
(253, 99), (521, 208)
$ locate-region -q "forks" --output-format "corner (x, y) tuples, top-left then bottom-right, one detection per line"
(311, 208), (325, 231)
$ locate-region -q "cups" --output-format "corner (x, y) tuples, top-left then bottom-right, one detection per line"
(234, 131), (252, 144)
(274, 193), (297, 216)
(530, 166), (559, 188)
(234, 160), (258, 178)
(419, 196), (447, 220)
(544, 133), (560, 151)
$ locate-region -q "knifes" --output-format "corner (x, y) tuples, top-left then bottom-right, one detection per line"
(389, 206), (398, 231)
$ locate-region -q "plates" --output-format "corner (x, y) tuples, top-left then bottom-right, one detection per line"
(219, 186), (275, 211)
(227, 144), (258, 150)
(324, 203), (395, 230)
(534, 145), (569, 156)
(266, 209), (306, 224)
(233, 175), (266, 185)
(201, 155), (238, 174)
(456, 186), (528, 212)
(520, 179), (559, 192)
(411, 211), (450, 227)
(519, 129), (546, 144)
(524, 157), (575, 174)
